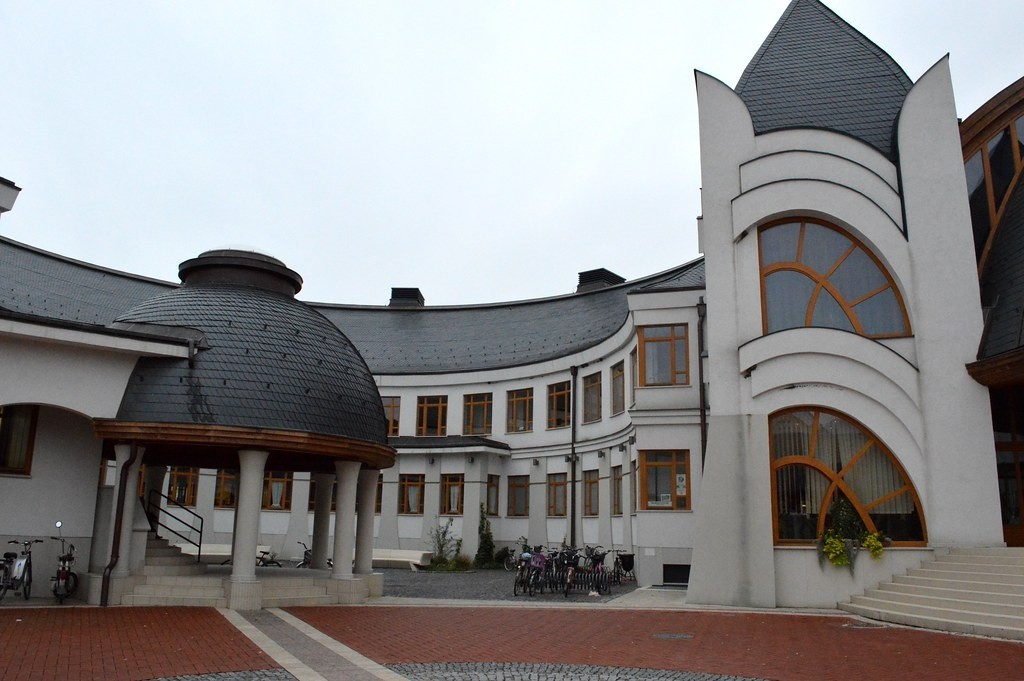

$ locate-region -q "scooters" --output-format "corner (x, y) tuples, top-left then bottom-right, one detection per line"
(0, 539), (44, 601)
(50, 520), (79, 605)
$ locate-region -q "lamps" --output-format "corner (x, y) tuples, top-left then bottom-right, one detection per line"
(619, 444), (626, 452)
(629, 436), (636, 445)
(533, 459), (539, 465)
(565, 456), (571, 462)
(598, 450), (605, 458)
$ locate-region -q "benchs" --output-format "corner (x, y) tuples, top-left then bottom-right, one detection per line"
(173, 544), (435, 572)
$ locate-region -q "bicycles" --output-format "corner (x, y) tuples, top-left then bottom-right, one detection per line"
(221, 551), (282, 568)
(295, 541), (333, 569)
(503, 536), (631, 598)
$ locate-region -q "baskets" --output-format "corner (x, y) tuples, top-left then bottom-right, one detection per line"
(585, 547), (595, 555)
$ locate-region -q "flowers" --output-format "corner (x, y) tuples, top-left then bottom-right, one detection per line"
(823, 538), (850, 566)
(863, 535), (882, 562)
